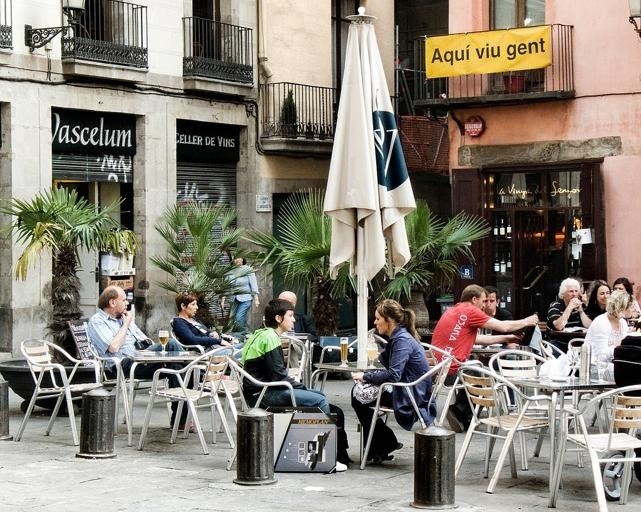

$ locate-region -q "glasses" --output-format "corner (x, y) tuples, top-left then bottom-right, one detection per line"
(182, 290), (194, 296)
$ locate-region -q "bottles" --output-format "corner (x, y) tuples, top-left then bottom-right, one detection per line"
(579, 344), (592, 382)
(492, 216), (512, 240)
(493, 250), (512, 274)
(571, 216), (577, 239)
(568, 258), (582, 280)
(521, 312), (539, 347)
(495, 182), (539, 209)
(499, 287), (512, 311)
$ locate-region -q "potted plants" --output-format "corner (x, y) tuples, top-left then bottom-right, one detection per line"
(149, 200), (259, 381)
(248, 186), (353, 379)
(368, 198), (493, 349)
(281, 89), (297, 138)
(1, 186), (142, 413)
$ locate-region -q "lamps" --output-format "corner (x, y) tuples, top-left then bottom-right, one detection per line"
(25, 0), (85, 53)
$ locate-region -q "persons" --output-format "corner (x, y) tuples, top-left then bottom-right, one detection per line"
(429, 283), (539, 432)
(172, 288), (245, 400)
(239, 297), (356, 469)
(86, 284), (206, 433)
(347, 298), (434, 468)
(217, 256), (261, 341)
(541, 275), (641, 386)
(261, 290), (341, 379)
(474, 286), (520, 406)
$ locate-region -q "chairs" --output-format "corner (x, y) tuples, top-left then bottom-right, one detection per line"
(23, 320), (640, 512)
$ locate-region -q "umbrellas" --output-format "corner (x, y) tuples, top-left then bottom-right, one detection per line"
(321, 16), (419, 371)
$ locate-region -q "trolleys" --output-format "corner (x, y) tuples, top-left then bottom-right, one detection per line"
(595, 334), (641, 504)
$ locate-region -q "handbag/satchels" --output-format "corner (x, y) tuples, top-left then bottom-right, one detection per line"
(353, 369), (393, 401)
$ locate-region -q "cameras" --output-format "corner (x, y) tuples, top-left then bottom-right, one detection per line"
(135, 339), (152, 349)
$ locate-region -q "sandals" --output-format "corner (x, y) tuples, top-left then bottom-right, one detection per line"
(372, 456), (393, 464)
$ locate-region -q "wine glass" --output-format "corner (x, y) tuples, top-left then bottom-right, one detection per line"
(339, 337), (348, 368)
(366, 344), (378, 368)
(157, 330), (170, 354)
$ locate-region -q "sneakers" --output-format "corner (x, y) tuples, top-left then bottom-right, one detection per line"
(446, 404), (466, 433)
(336, 460), (347, 471)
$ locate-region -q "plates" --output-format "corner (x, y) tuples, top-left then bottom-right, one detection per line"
(471, 345), (482, 349)
(549, 376), (567, 382)
